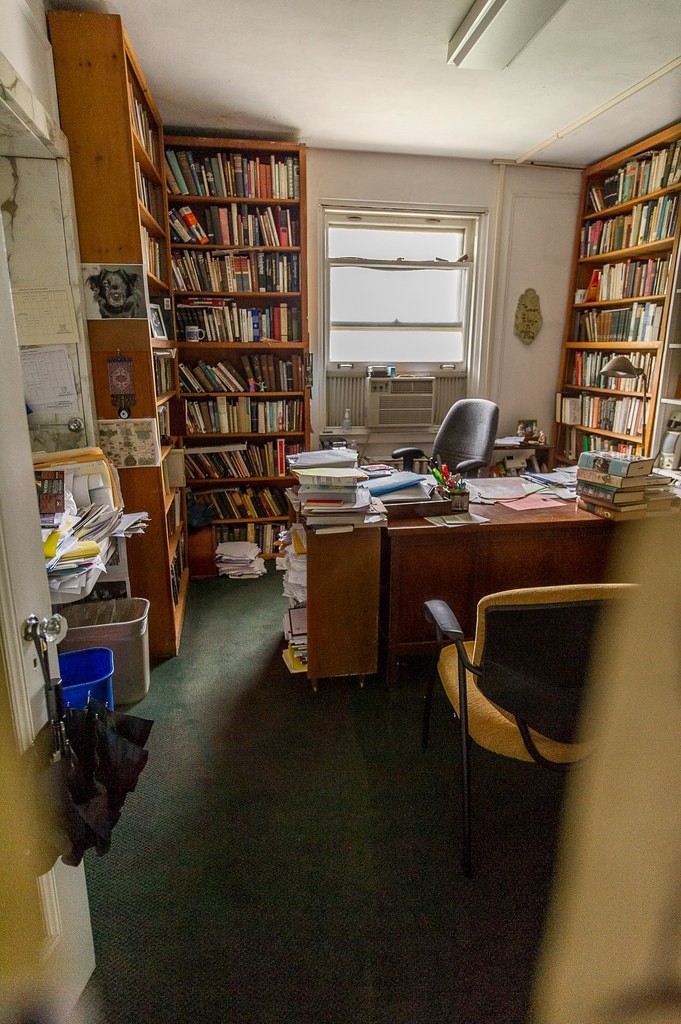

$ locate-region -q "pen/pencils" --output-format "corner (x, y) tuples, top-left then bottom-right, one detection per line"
(429, 454), (466, 491)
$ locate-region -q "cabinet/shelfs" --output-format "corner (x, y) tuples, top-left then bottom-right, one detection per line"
(650, 231), (681, 485)
(547, 119), (681, 470)
(283, 490), (388, 692)
(45, 9), (190, 658)
(162, 134), (311, 561)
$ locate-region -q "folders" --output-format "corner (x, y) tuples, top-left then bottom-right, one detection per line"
(358, 471), (429, 497)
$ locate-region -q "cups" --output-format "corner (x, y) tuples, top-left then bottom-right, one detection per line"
(575, 290), (587, 304)
(186, 326), (205, 342)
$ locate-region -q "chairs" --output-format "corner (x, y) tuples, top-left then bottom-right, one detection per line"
(391, 398), (499, 481)
(421, 583), (646, 875)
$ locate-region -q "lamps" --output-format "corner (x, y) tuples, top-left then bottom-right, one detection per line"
(598, 355), (648, 458)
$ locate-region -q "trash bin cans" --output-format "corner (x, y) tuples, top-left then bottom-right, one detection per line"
(58, 646), (115, 712)
(56, 597), (151, 706)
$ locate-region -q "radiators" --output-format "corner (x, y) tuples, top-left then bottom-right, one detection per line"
(326, 372), (467, 426)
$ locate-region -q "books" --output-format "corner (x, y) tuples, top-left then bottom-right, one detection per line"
(168, 203), (300, 246)
(169, 396), (304, 433)
(172, 249), (301, 293)
(572, 302), (663, 394)
(282, 608), (308, 673)
(179, 354), (304, 394)
(153, 347), (185, 608)
(575, 253), (672, 304)
(576, 450), (677, 521)
(186, 486), (289, 520)
(285, 469), (388, 534)
(555, 391), (651, 460)
(175, 297), (302, 343)
(164, 150), (299, 200)
(188, 523), (287, 554)
(128, 81), (162, 281)
(586, 138), (681, 217)
(580, 195), (681, 259)
(184, 439), (305, 480)
(34, 470), (124, 578)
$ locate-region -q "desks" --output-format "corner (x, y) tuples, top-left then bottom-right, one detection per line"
(385, 472), (616, 682)
